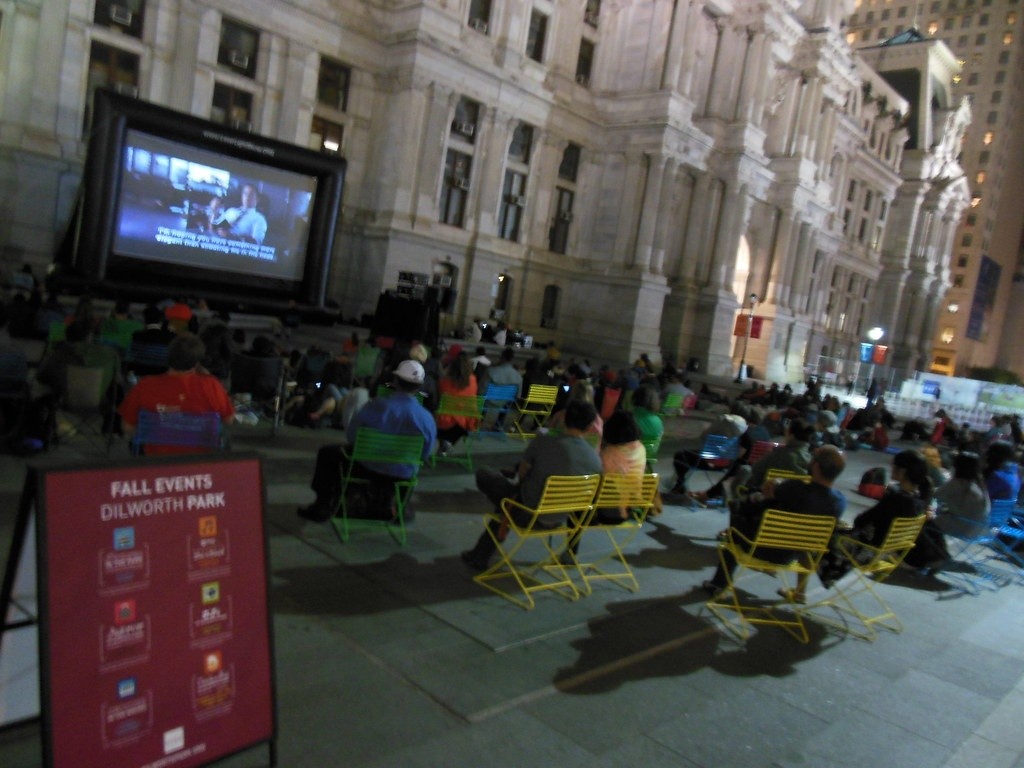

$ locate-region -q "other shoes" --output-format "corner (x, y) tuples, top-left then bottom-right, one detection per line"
(703, 580), (724, 597)
(690, 490), (709, 502)
(778, 587), (806, 603)
(297, 502), (331, 522)
(659, 491), (678, 502)
(462, 549), (488, 572)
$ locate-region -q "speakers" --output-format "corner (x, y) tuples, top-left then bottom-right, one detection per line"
(423, 287), (438, 307)
(439, 286), (457, 315)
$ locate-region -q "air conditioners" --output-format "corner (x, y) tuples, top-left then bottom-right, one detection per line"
(578, 75), (589, 86)
(227, 48), (248, 69)
(512, 196), (526, 207)
(586, 11), (598, 27)
(235, 120), (252, 133)
(562, 213), (573, 221)
(434, 275), (452, 286)
(471, 18), (487, 34)
(456, 122), (474, 136)
(455, 178), (470, 190)
(117, 82), (138, 98)
(491, 309), (506, 321)
(107, 5), (132, 26)
(543, 318), (556, 328)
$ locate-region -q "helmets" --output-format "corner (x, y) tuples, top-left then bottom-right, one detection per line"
(393, 360), (425, 385)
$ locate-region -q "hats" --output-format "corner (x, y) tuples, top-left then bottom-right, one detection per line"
(820, 410), (840, 434)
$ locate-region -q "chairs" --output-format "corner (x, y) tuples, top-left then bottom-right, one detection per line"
(49, 322), (69, 349)
(682, 394), (698, 416)
(469, 383), (519, 441)
(658, 393), (682, 422)
(668, 435), (743, 511)
(48, 359), (118, 449)
(224, 356), (289, 435)
(505, 385), (558, 442)
(100, 321), (144, 348)
(130, 342), (171, 366)
(926, 433), (1024, 596)
(748, 441), (787, 465)
(706, 511), (927, 645)
(132, 411), (222, 458)
(735, 471), (810, 502)
(377, 387), (424, 407)
(424, 394), (486, 471)
(333, 426), (423, 544)
(351, 348), (379, 392)
(473, 474), (660, 611)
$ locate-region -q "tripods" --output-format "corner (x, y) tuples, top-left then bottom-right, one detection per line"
(437, 315), (449, 356)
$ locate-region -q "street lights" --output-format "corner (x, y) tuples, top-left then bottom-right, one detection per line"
(734, 293), (757, 385)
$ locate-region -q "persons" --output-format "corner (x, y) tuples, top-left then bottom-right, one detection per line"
(982, 442), (1021, 512)
(460, 401), (601, 572)
(932, 453), (991, 574)
(296, 359), (438, 523)
(0, 260), (1024, 508)
(189, 185), (270, 246)
(704, 443), (845, 599)
(824, 450), (929, 587)
(560, 408), (648, 568)
(117, 335), (237, 460)
